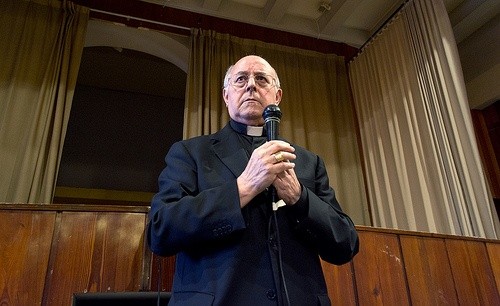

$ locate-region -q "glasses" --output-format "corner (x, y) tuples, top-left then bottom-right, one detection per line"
(227, 73), (278, 87)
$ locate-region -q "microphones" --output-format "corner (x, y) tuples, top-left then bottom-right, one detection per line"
(263, 104), (282, 197)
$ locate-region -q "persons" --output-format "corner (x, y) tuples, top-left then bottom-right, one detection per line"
(143, 54), (360, 306)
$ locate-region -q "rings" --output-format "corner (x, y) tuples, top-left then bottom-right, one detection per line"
(274, 151), (283, 161)
